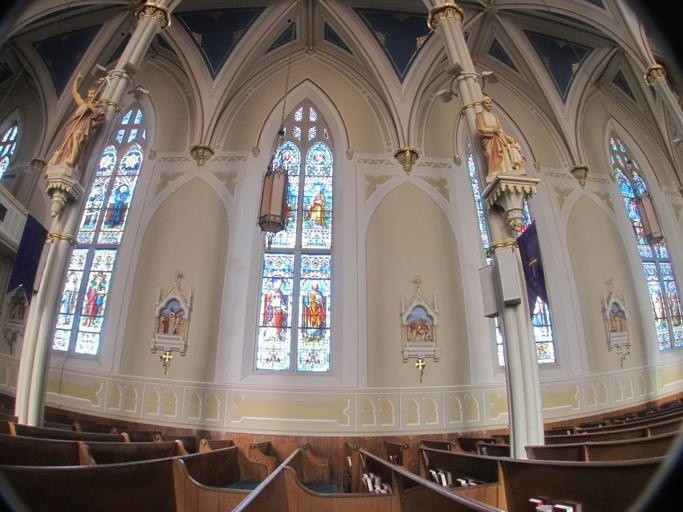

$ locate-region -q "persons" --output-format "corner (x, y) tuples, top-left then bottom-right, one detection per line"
(47, 73), (107, 171)
(306, 184), (327, 226)
(58, 274), (78, 326)
(303, 283), (325, 343)
(107, 186), (129, 230)
(474, 96), (530, 184)
(83, 276), (107, 327)
(258, 278), (289, 341)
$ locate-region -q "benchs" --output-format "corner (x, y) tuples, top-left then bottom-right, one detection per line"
(1, 393), (683, 512)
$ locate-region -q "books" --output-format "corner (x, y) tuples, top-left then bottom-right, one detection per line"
(346, 446), (575, 512)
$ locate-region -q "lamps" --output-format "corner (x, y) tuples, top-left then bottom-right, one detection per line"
(542, 1), (664, 245)
(257, 3), (300, 249)
